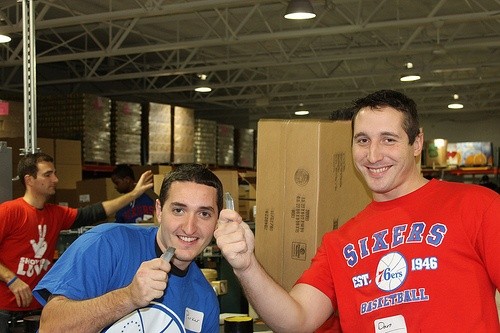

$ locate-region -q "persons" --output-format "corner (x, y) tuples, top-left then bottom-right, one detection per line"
(34, 166), (226, 333)
(111, 164), (158, 223)
(212, 88), (500, 333)
(0, 151), (155, 333)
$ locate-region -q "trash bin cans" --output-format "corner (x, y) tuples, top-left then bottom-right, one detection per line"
(223, 315), (254, 333)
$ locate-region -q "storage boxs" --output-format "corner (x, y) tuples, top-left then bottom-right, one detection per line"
(0, 91), (372, 323)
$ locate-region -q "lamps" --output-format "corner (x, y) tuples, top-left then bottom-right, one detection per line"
(195, 74), (213, 92)
(284, 0), (316, 20)
(399, 64), (421, 82)
(294, 102), (309, 116)
(447, 94), (464, 109)
